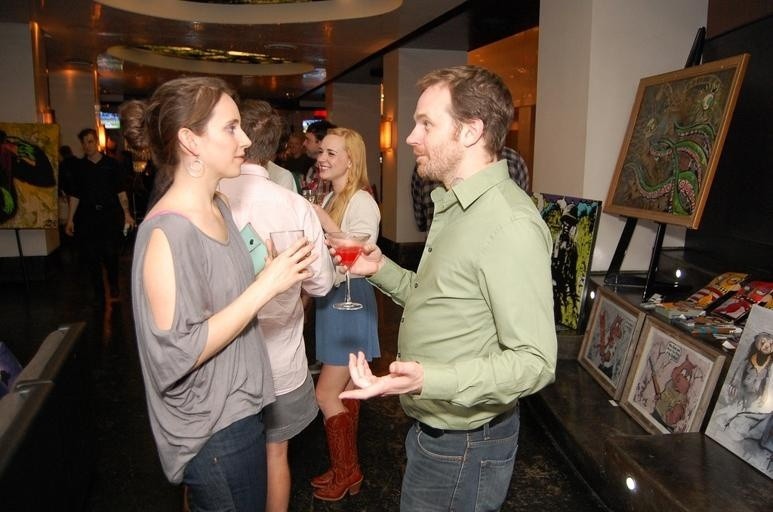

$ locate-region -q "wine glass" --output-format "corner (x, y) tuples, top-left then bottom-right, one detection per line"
(322, 230), (372, 310)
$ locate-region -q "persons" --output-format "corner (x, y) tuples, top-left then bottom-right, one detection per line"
(409, 144), (532, 251)
(213, 98), (334, 511)
(310, 126), (382, 502)
(121, 77), (317, 510)
(237, 95), (323, 375)
(550, 203), (579, 327)
(53, 97), (159, 381)
(327, 65), (557, 512)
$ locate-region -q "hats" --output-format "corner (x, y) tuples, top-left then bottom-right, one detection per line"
(560, 204), (578, 223)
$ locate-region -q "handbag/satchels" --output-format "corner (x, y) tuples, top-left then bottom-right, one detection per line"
(238, 223), (269, 277)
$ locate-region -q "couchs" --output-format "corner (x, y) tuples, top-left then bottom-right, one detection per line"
(1, 318), (110, 510)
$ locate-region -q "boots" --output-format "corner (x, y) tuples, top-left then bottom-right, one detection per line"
(313, 409), (364, 502)
(310, 399), (362, 489)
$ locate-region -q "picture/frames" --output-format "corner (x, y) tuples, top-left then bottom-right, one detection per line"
(602, 52), (751, 231)
(578, 286), (645, 399)
(620, 315), (727, 435)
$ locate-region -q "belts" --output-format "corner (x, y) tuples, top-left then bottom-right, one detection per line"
(93, 205), (101, 209)
(413, 412), (515, 439)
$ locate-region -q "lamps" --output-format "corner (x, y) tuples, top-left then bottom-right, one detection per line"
(380, 119), (393, 150)
(98, 124), (107, 150)
(41, 107), (55, 125)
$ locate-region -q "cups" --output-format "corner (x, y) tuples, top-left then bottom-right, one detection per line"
(301, 189), (317, 205)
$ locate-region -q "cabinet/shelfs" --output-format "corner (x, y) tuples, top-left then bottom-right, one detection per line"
(525, 242), (772, 512)
